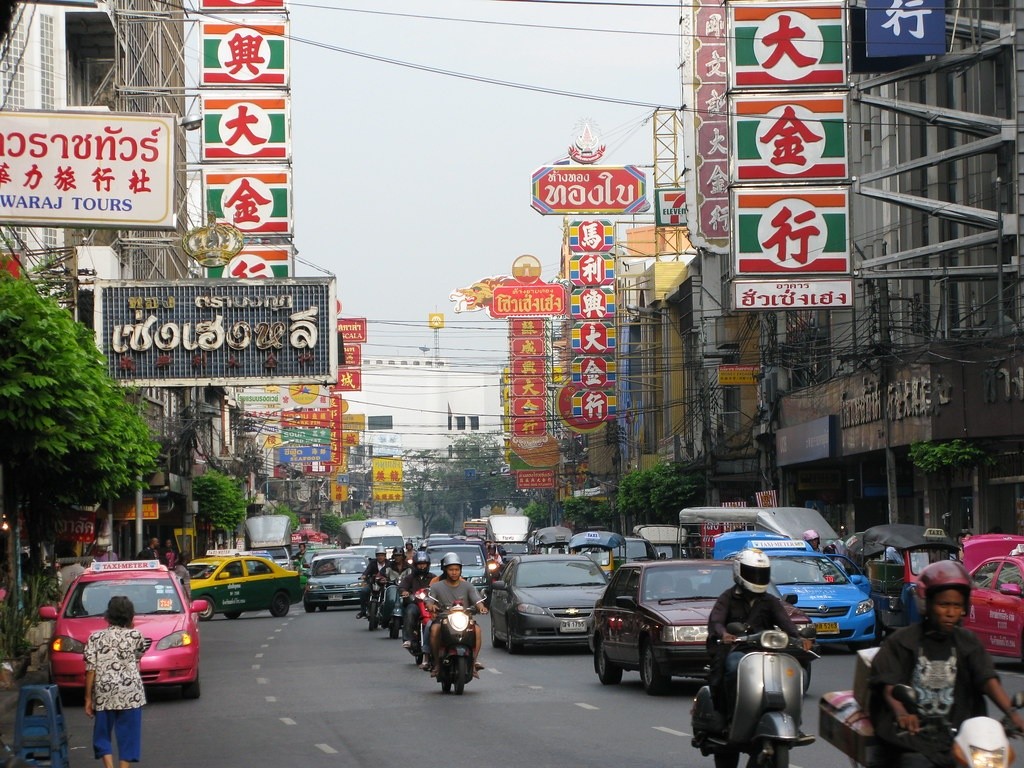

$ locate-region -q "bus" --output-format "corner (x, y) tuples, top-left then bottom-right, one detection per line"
(463, 518), (488, 538)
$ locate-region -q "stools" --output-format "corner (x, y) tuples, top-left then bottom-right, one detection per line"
(13, 683), (68, 766)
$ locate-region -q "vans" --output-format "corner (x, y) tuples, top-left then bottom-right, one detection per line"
(359, 520), (406, 560)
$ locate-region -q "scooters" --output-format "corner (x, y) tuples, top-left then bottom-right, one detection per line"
(691, 619), (817, 767)
(354, 577), (434, 667)
(849, 681), (1024, 767)
(423, 587), (488, 696)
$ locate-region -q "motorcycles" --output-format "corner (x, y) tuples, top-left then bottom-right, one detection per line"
(339, 520), (366, 549)
(843, 522), (963, 633)
(533, 524), (692, 580)
(676, 505), (846, 567)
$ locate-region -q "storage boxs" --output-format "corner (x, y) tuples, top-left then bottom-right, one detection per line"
(819, 647), (882, 767)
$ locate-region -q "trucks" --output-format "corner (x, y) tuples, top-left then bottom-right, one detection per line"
(245, 513), (293, 572)
(488, 515), (529, 564)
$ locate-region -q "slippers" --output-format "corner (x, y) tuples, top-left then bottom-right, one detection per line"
(476, 661), (484, 670)
(419, 661), (430, 669)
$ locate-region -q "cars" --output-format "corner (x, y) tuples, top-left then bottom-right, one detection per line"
(586, 558), (819, 694)
(186, 550), (303, 621)
(39, 559), (209, 707)
(254, 540), (378, 592)
(403, 533), (489, 570)
(960, 533), (1024, 669)
(491, 553), (614, 655)
(712, 532), (876, 653)
(422, 544), (492, 613)
(303, 554), (369, 613)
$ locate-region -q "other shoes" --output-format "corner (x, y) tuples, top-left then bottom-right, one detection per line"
(402, 640), (411, 648)
(474, 671), (480, 679)
(356, 611), (365, 619)
(431, 665), (440, 678)
(793, 731), (815, 747)
(382, 617), (388, 629)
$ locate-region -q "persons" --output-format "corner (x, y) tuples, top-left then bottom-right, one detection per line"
(405, 542), (417, 561)
(419, 552), (488, 677)
(883, 546), (904, 564)
(377, 546), (413, 628)
(485, 541), (502, 565)
(707, 548), (816, 745)
(82, 596), (147, 768)
(60, 551), (84, 601)
(135, 537), (177, 566)
(398, 551), (436, 647)
(175, 551), (191, 600)
(870, 560), (1024, 768)
(951, 526), (1003, 562)
(803, 530), (845, 571)
(356, 546), (390, 620)
(296, 543), (306, 555)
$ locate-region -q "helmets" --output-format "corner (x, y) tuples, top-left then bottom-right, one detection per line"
(392, 546), (406, 559)
(406, 542), (413, 550)
(488, 544), (496, 553)
(443, 553), (462, 568)
(375, 546), (386, 557)
(441, 552), (455, 569)
(917, 560), (971, 616)
(420, 543), (427, 549)
(804, 529), (820, 545)
(732, 548), (771, 593)
(411, 551), (431, 575)
